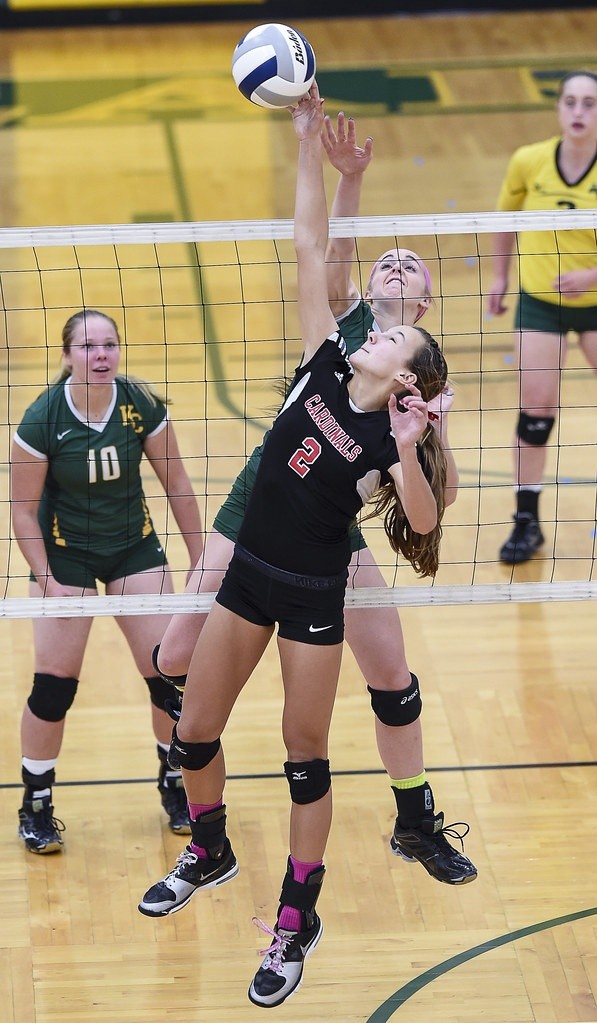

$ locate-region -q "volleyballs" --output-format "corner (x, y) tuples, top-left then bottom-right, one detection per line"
(229, 22), (317, 112)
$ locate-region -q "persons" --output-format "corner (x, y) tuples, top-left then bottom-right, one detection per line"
(138, 79), (448, 1007)
(10, 310), (204, 855)
(153, 112), (478, 885)
(485, 69), (597, 565)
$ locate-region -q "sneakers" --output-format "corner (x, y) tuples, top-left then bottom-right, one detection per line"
(17, 805), (66, 854)
(390, 811), (478, 886)
(136, 837), (240, 918)
(248, 909), (323, 1008)
(162, 787), (193, 835)
(501, 514), (545, 565)
